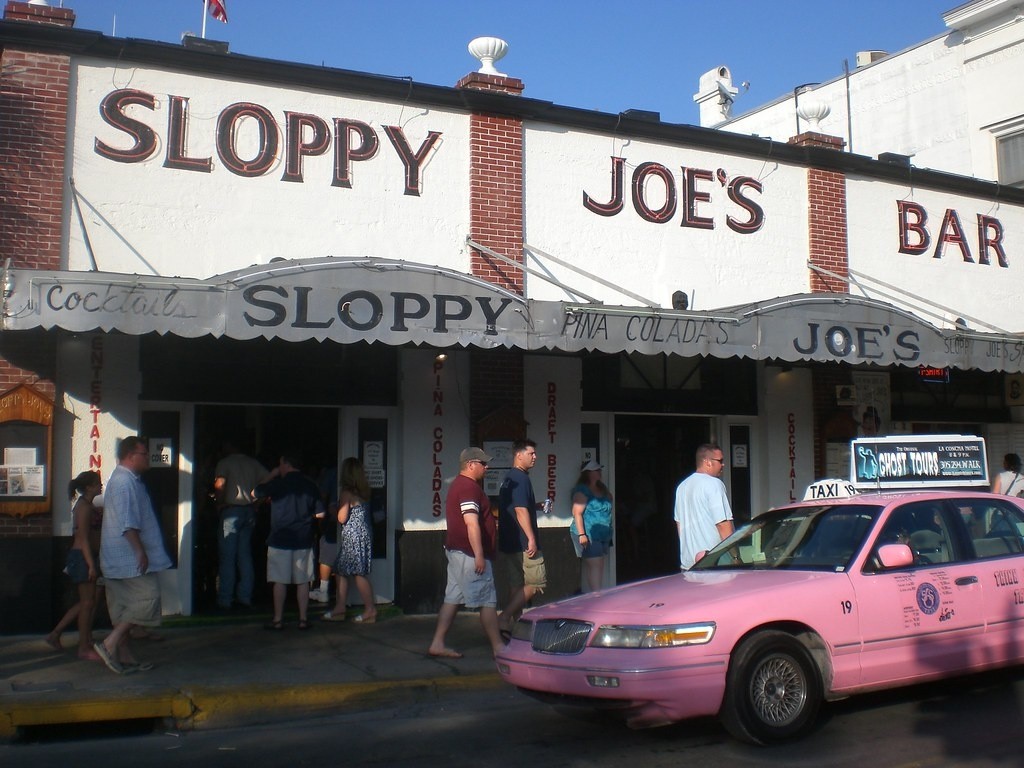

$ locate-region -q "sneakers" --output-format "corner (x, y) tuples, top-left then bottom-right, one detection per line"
(309, 588), (329, 603)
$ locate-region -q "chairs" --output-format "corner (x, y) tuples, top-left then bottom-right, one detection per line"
(907, 530), (945, 564)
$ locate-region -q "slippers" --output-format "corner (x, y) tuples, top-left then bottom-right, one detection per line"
(498, 623), (512, 643)
(427, 647), (464, 658)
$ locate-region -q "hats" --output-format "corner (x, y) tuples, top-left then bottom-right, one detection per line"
(460, 447), (494, 462)
(580, 460), (605, 472)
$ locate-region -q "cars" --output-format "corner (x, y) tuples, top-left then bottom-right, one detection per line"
(496, 492), (1024, 746)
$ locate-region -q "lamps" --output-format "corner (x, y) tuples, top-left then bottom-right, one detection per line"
(435, 352), (447, 362)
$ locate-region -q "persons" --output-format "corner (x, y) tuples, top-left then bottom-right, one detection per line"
(992, 453), (1024, 498)
(570, 460), (614, 592)
(674, 444), (743, 572)
(426, 447), (509, 661)
(496, 438), (553, 642)
(45, 471), (103, 661)
(93, 436), (173, 677)
(880, 513), (920, 563)
(206, 437), (377, 631)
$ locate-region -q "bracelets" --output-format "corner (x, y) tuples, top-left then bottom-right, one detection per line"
(733, 555), (741, 561)
(579, 534), (586, 537)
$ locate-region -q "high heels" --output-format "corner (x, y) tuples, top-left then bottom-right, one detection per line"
(351, 614), (377, 624)
(321, 611), (345, 622)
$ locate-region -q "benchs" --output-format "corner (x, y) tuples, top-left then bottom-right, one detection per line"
(973, 535), (1024, 557)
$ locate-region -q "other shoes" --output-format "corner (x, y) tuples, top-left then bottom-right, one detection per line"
(93, 642), (154, 675)
(130, 630), (163, 642)
(75, 645), (98, 660)
(295, 621), (311, 630)
(263, 620), (284, 630)
(217, 594), (251, 607)
(46, 631), (65, 651)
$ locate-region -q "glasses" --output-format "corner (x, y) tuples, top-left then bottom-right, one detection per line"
(710, 458), (725, 464)
(134, 451), (150, 458)
(467, 460), (486, 466)
